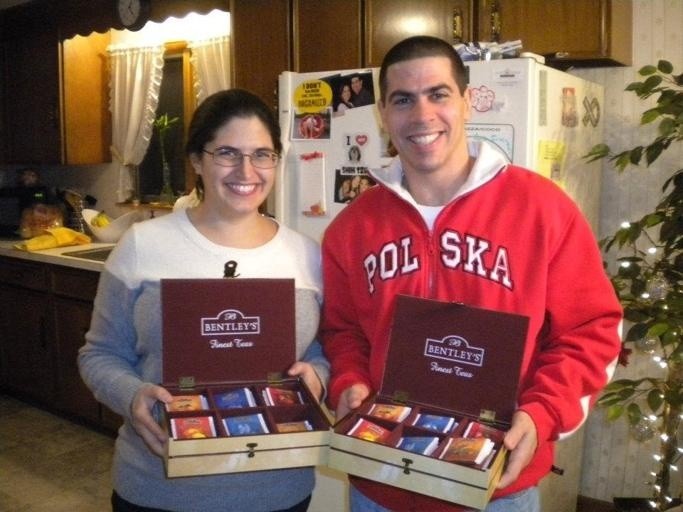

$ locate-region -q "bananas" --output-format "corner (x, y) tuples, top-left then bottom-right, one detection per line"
(92, 211), (109, 227)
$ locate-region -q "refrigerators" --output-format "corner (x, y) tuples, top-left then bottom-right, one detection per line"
(270, 50), (606, 511)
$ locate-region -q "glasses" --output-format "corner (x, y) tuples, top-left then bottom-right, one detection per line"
(201, 146), (279, 169)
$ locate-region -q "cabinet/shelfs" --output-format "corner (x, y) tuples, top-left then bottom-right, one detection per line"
(0, 24), (112, 169)
(0, 254), (124, 440)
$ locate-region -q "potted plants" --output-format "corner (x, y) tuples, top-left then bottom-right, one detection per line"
(581, 60), (683, 512)
(153, 112), (180, 205)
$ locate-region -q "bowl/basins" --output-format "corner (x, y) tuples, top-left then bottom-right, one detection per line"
(79, 206), (138, 244)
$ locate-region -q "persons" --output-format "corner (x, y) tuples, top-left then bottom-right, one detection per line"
(348, 147), (362, 164)
(341, 175), (361, 198)
(315, 35), (623, 511)
(334, 83), (353, 112)
(348, 73), (376, 105)
(335, 180), (350, 203)
(357, 175), (373, 194)
(302, 113), (319, 140)
(75, 87), (330, 511)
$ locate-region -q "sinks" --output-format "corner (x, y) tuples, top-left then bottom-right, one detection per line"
(58, 244), (116, 265)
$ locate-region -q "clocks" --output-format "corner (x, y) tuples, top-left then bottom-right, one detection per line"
(114, 0), (150, 31)
